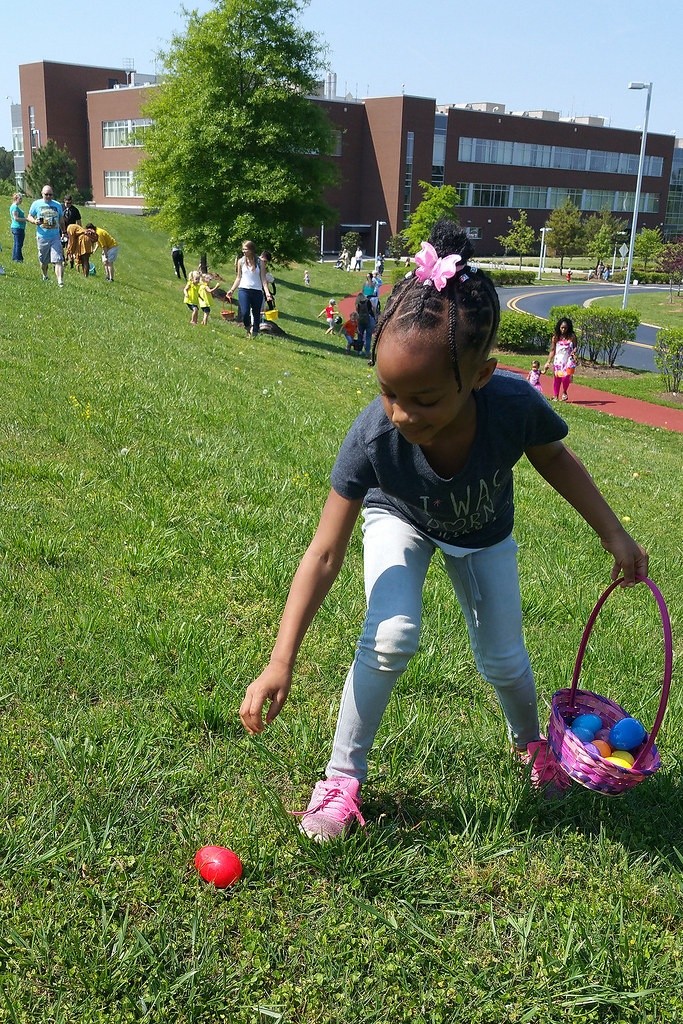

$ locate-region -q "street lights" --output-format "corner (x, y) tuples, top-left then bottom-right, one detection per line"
(375, 220), (387, 271)
(611, 231), (627, 275)
(622, 82), (654, 308)
(538, 227), (552, 280)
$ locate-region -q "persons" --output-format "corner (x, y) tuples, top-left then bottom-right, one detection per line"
(238, 222), (651, 846)
(404, 258), (410, 268)
(377, 253), (384, 270)
(226, 240), (273, 340)
(340, 249), (349, 268)
(526, 361), (549, 393)
(59, 194), (119, 283)
(183, 270), (220, 325)
(10, 192), (29, 263)
(172, 247), (188, 282)
(317, 299), (340, 335)
(545, 317), (581, 402)
(354, 246), (363, 271)
(303, 270), (310, 286)
(337, 270), (382, 359)
(27, 186), (68, 288)
(0, 241), (6, 275)
(623, 263), (628, 271)
(597, 262), (610, 280)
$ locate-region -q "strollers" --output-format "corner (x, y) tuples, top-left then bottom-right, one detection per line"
(335, 258), (346, 270)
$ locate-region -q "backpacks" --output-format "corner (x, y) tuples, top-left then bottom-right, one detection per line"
(357, 299), (370, 326)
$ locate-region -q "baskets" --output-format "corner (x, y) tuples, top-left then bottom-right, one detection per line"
(547, 573), (673, 798)
(220, 297), (235, 322)
(353, 333), (365, 353)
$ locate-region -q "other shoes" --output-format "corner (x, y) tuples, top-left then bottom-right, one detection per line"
(43, 273), (49, 282)
(552, 398), (559, 401)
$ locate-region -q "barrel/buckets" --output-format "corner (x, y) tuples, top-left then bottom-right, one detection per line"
(88, 262), (96, 274)
(263, 300), (278, 321)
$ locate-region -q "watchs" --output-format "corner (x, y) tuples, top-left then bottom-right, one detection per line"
(61, 231), (67, 234)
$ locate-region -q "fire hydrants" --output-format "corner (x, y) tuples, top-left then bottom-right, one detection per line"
(565, 268), (573, 283)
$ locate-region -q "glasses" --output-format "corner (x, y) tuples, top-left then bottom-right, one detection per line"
(43, 192), (53, 196)
(66, 202), (72, 205)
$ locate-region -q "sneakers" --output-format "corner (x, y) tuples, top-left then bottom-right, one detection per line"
(562, 391), (568, 401)
(509, 733), (568, 796)
(299, 776), (362, 846)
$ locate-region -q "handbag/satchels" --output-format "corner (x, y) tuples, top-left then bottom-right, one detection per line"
(335, 314), (342, 324)
(89, 261), (96, 276)
(264, 300), (278, 321)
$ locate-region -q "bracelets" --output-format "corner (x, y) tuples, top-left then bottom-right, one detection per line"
(230, 289), (234, 292)
